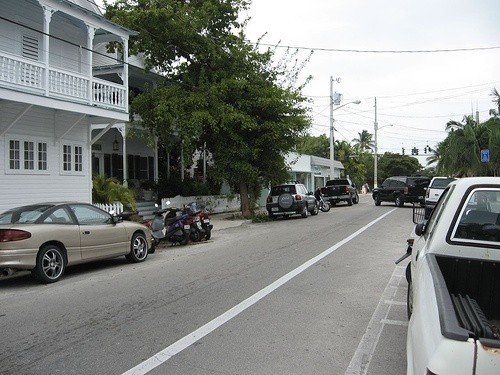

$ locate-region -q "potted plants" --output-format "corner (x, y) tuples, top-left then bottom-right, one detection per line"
(140, 180), (156, 201)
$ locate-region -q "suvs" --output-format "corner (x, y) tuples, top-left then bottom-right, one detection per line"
(265, 180), (319, 219)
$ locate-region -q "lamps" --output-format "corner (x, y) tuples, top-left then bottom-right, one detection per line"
(113, 135), (119, 150)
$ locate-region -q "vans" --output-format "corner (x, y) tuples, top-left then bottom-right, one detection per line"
(372, 175), (430, 207)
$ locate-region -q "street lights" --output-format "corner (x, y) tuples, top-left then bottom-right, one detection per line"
(330, 100), (362, 180)
(373, 124), (394, 189)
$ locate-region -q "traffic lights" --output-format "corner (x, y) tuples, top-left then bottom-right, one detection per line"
(424, 148), (427, 154)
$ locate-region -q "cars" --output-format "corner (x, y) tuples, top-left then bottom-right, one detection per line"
(0, 201), (153, 284)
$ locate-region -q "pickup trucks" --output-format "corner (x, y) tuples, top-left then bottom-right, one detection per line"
(424, 176), (461, 220)
(321, 178), (360, 206)
(407, 176), (500, 375)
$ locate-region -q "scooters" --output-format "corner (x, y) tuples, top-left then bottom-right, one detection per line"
(309, 189), (331, 212)
(116, 199), (213, 246)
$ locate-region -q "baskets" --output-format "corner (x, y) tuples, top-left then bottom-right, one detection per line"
(412, 203), (435, 225)
